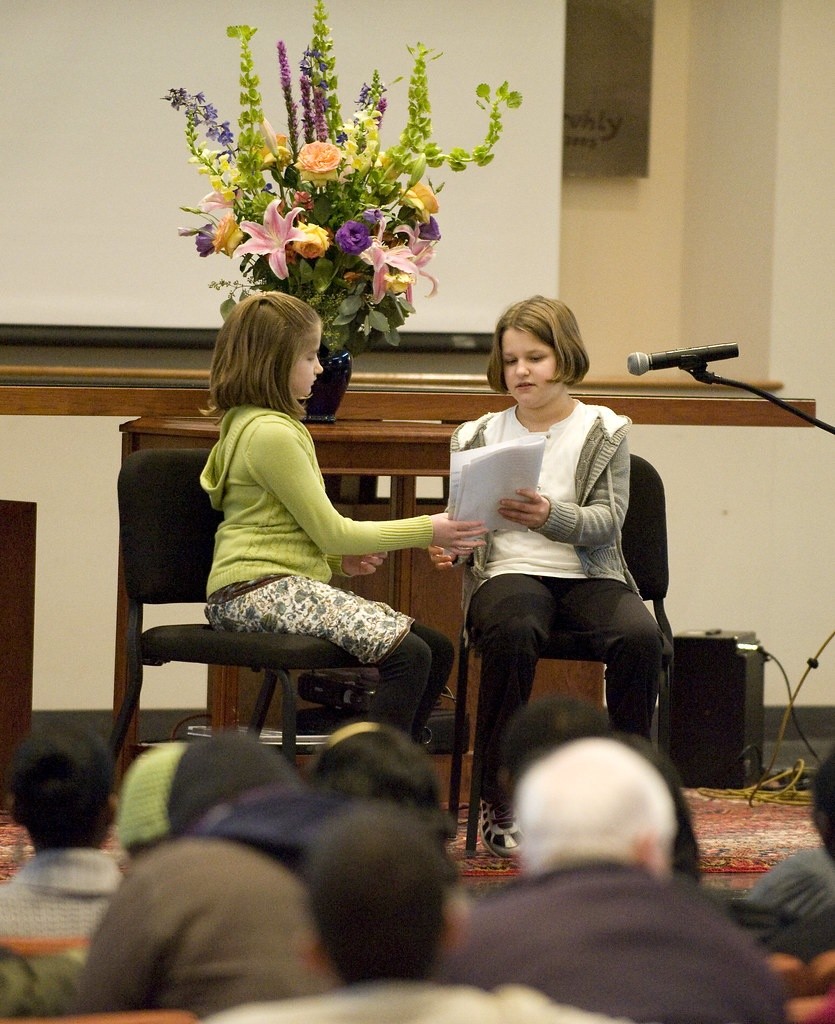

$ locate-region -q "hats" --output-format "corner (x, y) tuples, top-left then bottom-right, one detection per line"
(167, 734), (298, 836)
(119, 743), (194, 851)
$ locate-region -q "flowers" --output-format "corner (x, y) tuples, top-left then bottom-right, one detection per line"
(157, 0), (522, 352)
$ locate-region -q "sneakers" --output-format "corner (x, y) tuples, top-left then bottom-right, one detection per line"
(481, 797), (524, 860)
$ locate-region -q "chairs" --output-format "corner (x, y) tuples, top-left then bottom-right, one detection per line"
(446, 453), (674, 859)
(101, 446), (381, 772)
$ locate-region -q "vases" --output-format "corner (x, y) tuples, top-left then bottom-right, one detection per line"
(297, 347), (354, 424)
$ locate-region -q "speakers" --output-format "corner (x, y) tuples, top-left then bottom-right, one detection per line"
(671, 628), (766, 789)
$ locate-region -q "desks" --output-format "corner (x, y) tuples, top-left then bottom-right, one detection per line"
(115, 418), (605, 820)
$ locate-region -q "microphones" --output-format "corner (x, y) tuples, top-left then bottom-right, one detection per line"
(627, 342), (740, 376)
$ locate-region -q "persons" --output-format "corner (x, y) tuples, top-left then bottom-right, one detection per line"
(197, 289), (489, 746)
(427, 294), (666, 862)
(0, 695), (835, 1024)
